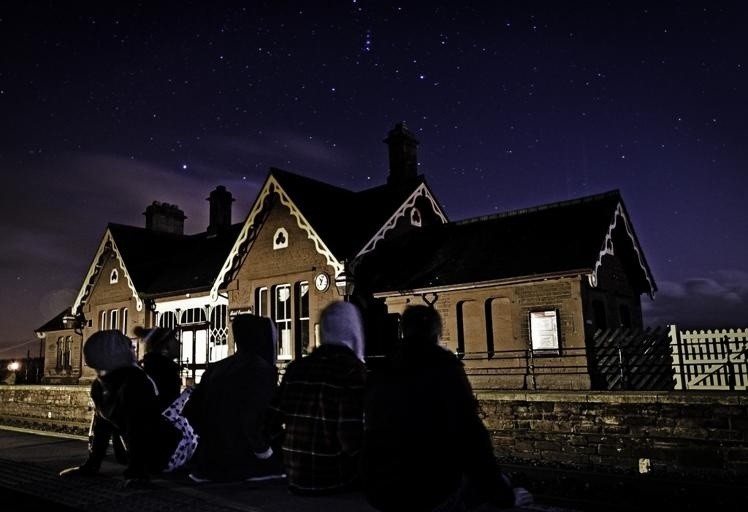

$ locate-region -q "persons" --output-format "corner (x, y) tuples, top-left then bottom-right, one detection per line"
(180, 313), (289, 480)
(273, 299), (376, 494)
(369, 305), (536, 511)
(132, 321), (183, 406)
(56, 329), (200, 487)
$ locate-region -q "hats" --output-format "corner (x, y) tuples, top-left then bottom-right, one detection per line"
(84, 330), (132, 368)
(135, 327), (171, 351)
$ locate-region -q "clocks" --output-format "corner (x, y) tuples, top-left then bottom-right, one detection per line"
(312, 271), (331, 295)
